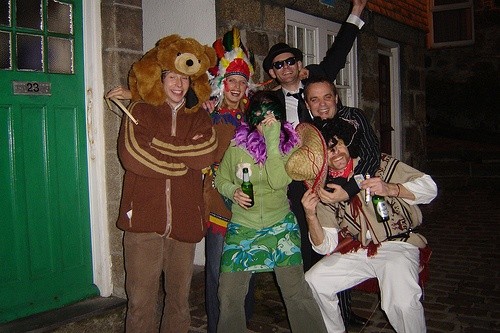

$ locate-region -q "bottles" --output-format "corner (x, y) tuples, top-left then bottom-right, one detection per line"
(370, 172), (389, 223)
(241, 168), (255, 207)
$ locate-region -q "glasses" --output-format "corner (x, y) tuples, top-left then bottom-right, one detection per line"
(272, 56), (296, 69)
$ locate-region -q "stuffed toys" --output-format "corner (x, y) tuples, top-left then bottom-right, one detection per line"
(128, 33), (217, 113)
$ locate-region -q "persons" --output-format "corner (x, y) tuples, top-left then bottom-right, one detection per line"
(247, 0), (367, 273)
(116, 68), (219, 333)
(213, 89), (328, 333)
(106, 73), (254, 333)
(301, 119), (438, 333)
(303, 77), (381, 333)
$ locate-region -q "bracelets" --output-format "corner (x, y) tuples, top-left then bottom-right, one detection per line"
(396, 184), (401, 197)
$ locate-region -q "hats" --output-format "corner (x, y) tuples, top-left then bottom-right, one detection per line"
(282, 121), (328, 205)
(262, 42), (304, 76)
